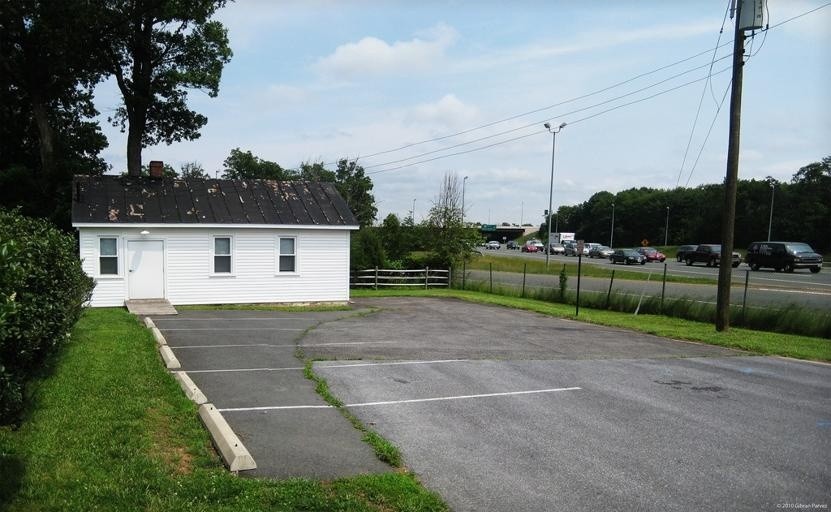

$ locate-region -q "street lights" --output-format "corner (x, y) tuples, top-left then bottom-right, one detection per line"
(545, 121), (566, 266)
(610, 204), (616, 248)
(768, 184), (775, 242)
(664, 206), (669, 247)
(461, 176), (468, 226)
(413, 198), (417, 225)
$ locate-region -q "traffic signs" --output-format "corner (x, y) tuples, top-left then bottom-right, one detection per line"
(480, 225), (496, 232)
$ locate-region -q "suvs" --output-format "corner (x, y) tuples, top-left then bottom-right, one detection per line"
(745, 242), (823, 273)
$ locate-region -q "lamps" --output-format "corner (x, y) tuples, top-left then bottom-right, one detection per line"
(139, 230), (150, 236)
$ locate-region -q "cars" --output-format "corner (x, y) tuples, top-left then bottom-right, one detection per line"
(544, 232), (615, 259)
(608, 246), (667, 265)
(506, 240), (544, 253)
(479, 240), (500, 249)
(675, 243), (742, 268)
